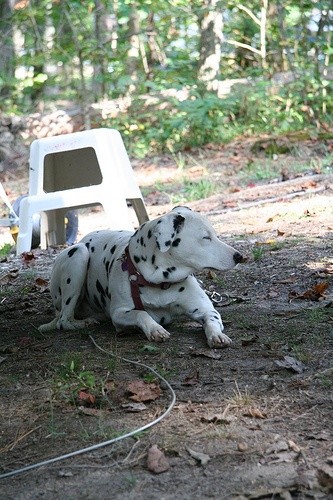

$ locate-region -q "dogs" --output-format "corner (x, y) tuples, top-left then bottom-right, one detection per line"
(38, 206), (248, 350)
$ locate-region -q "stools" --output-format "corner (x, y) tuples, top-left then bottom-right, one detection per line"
(12, 122), (155, 252)
